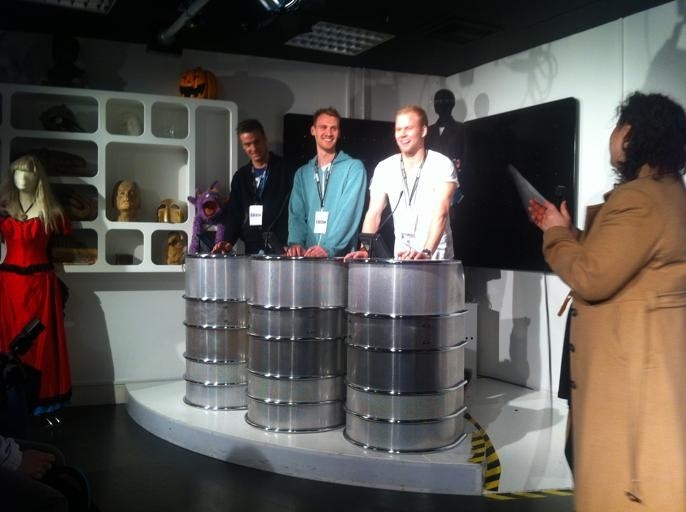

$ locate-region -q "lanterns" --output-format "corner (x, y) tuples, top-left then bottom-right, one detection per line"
(177, 67), (221, 99)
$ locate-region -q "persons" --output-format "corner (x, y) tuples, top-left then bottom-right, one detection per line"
(425, 87), (466, 162)
(211, 120), (297, 255)
(0, 152), (72, 417)
(526, 91), (686, 512)
(113, 178), (140, 221)
(0, 435), (73, 512)
(285, 107), (367, 258)
(342, 106), (460, 264)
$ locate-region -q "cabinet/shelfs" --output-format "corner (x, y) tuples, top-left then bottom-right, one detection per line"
(0, 82), (240, 275)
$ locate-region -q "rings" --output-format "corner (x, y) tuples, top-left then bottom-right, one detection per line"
(308, 247), (313, 250)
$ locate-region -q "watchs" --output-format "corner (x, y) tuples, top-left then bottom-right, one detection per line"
(420, 249), (431, 257)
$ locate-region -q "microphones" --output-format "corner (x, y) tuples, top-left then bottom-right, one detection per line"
(197, 209), (231, 254)
(368, 190), (404, 261)
(263, 191), (292, 255)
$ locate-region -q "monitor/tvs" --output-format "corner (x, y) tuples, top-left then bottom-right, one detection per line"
(262, 231), (286, 255)
(359, 232), (394, 258)
(453, 97), (581, 275)
(282, 112), (395, 223)
